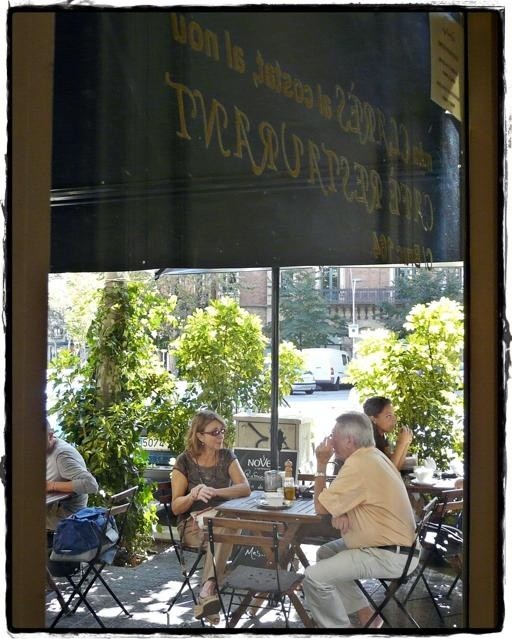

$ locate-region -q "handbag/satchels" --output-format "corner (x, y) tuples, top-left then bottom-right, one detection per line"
(50, 506), (119, 562)
(190, 507), (223, 530)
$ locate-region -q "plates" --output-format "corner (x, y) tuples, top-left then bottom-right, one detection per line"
(257, 499), (292, 508)
(409, 470), (438, 485)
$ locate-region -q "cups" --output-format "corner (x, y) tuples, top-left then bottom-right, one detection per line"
(265, 492), (283, 504)
(265, 470), (283, 491)
(284, 487), (295, 499)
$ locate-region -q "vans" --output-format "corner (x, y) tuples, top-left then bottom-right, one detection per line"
(301, 348), (357, 390)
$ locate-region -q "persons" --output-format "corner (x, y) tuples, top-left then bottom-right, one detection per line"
(302, 410), (422, 628)
(45, 418), (101, 532)
(335, 395), (416, 477)
(170, 409), (253, 624)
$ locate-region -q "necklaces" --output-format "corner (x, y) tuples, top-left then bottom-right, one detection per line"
(195, 451), (218, 485)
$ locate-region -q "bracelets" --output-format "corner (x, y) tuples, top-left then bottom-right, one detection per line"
(313, 472), (326, 478)
(53, 480), (55, 493)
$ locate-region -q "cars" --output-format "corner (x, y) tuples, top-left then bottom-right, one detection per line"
(258, 366), (317, 395)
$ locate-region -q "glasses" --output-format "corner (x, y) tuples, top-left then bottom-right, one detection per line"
(200, 429), (226, 436)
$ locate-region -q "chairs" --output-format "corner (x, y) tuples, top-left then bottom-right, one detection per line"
(154, 469), (467, 629)
(44, 486), (141, 629)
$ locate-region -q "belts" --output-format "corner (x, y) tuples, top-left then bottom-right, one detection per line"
(378, 545), (419, 557)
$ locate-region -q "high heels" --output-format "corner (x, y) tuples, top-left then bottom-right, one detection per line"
(193, 595), (221, 624)
